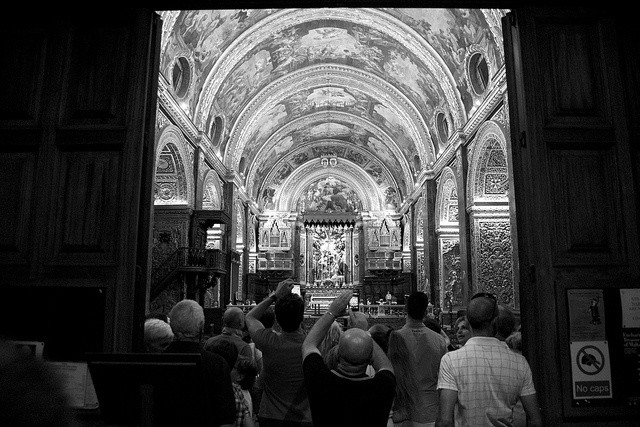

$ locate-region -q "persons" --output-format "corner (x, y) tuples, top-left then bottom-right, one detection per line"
(208, 338), (252, 425)
(453, 318), (470, 346)
(232, 352), (257, 422)
(368, 321), (394, 344)
(144, 309), (174, 354)
(204, 306), (251, 382)
(320, 323), (341, 368)
(425, 316), (442, 333)
(141, 300), (237, 427)
(438, 296), (543, 425)
(301, 291), (397, 424)
(388, 290), (447, 427)
(251, 311), (282, 412)
(498, 306), (522, 354)
(246, 279), (312, 425)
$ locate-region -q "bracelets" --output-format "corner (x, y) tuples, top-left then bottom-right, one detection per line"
(325, 311), (336, 320)
(267, 291), (277, 302)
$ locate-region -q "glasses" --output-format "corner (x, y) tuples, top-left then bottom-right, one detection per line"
(471, 292), (498, 314)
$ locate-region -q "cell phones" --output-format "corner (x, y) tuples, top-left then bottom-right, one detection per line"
(348, 293), (360, 312)
(290, 283), (301, 296)
